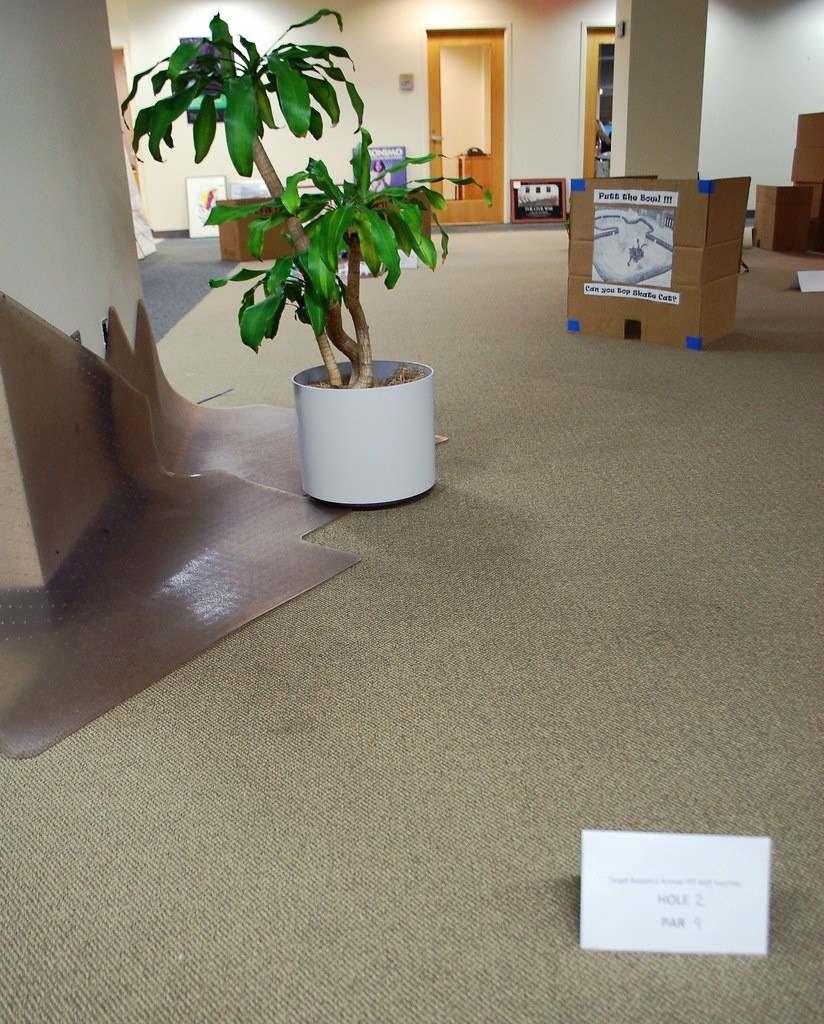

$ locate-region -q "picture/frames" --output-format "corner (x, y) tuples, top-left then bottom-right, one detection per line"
(510, 179), (566, 224)
(184, 175), (228, 239)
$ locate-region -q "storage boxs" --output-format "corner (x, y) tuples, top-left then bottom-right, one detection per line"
(790, 112), (824, 253)
(216, 198), (293, 262)
(751, 185), (814, 253)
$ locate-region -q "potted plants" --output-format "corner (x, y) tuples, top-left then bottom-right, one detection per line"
(119, 8), (437, 510)
(566, 175), (751, 351)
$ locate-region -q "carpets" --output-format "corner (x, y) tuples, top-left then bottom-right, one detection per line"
(138, 237), (240, 343)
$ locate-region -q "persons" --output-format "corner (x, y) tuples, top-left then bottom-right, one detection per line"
(369, 160), (391, 193)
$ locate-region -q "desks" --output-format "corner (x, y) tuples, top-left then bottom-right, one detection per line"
(454, 154), (489, 200)
